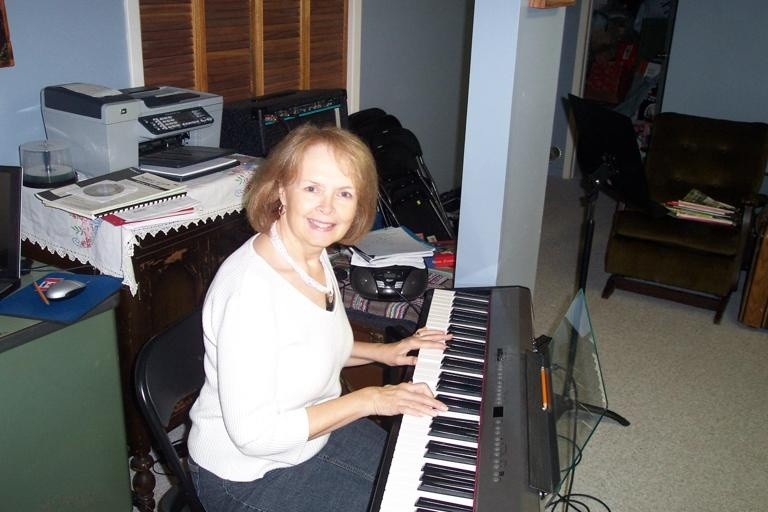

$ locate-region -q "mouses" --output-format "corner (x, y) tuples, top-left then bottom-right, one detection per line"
(45, 280), (87, 301)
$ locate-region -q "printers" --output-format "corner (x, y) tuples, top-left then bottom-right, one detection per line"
(41, 79), (241, 182)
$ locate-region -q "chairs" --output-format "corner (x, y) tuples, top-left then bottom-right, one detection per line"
(347, 108), (456, 242)
(601, 111), (768, 325)
(136, 309), (210, 511)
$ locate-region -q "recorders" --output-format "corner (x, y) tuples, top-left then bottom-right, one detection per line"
(350, 262), (429, 302)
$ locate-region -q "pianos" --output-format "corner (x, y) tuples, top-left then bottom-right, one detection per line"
(366, 286), (561, 511)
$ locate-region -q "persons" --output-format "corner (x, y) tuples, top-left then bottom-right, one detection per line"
(185, 122), (453, 511)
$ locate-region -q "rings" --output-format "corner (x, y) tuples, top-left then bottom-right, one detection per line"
(416, 331), (421, 335)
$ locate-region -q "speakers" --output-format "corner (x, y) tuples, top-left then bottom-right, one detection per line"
(222, 86), (348, 159)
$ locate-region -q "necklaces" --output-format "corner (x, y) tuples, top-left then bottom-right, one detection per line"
(270, 219), (334, 297)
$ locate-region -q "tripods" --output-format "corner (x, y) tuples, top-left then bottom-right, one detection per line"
(553, 182), (630, 427)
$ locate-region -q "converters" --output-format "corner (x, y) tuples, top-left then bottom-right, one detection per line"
(334, 267), (348, 280)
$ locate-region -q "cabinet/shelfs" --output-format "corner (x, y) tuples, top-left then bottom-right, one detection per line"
(0, 257), (133, 512)
(20, 155), (257, 512)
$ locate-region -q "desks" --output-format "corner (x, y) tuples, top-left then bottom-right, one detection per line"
(737, 226), (768, 329)
(336, 267), (453, 428)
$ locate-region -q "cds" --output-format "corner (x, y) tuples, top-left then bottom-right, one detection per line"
(24, 164), (75, 184)
(83, 184), (124, 197)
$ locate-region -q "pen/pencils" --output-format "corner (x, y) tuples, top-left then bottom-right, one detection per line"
(540, 366), (547, 409)
(33, 281), (50, 305)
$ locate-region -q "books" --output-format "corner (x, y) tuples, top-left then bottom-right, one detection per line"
(34, 166), (201, 226)
(430, 240), (456, 269)
(342, 224), (437, 269)
(659, 188), (738, 227)
